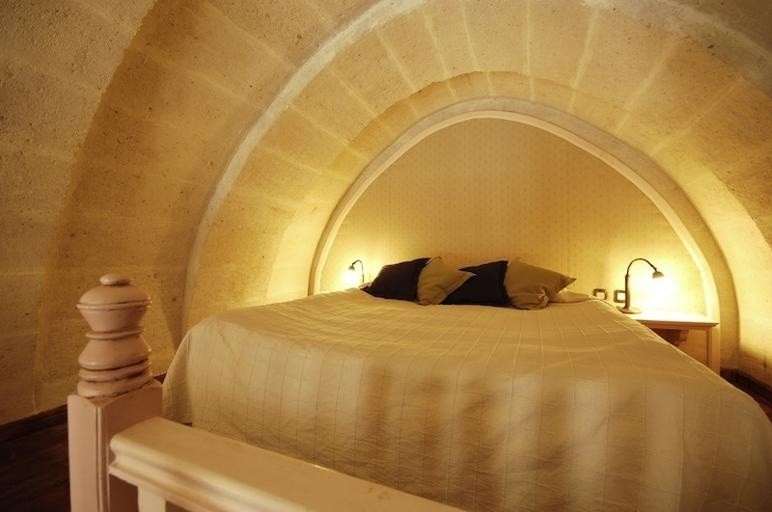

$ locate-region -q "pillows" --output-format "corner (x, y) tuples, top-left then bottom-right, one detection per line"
(360, 256), (430, 301)
(503, 255), (578, 310)
(414, 254), (477, 304)
(447, 259), (510, 309)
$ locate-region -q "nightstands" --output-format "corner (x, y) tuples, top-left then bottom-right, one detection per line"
(635, 319), (718, 372)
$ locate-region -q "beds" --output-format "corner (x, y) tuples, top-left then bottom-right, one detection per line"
(67, 252), (771, 512)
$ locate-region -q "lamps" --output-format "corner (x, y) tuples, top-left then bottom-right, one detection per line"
(612, 255), (667, 315)
(345, 259), (365, 285)
